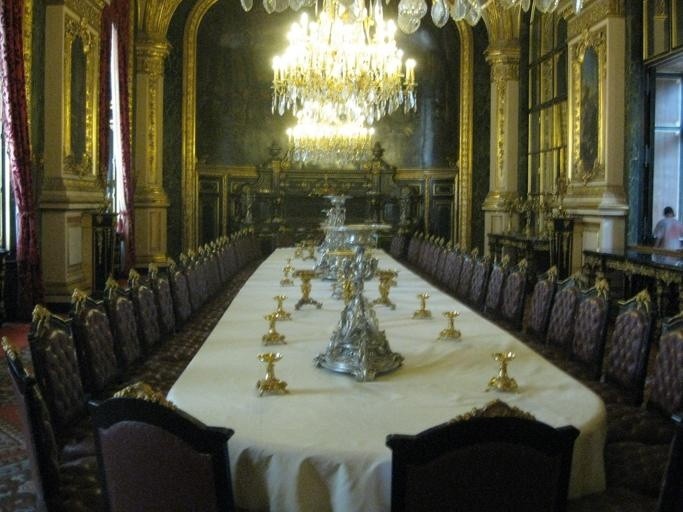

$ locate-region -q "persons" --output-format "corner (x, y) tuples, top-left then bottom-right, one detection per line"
(651, 207), (683, 265)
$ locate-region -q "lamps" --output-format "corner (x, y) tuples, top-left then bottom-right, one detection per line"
(268, 0), (419, 127)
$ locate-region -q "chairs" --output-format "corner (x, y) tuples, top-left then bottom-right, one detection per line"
(0, 224), (683, 511)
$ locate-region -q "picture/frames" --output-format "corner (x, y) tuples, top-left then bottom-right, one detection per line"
(568, 23), (606, 187)
(62, 11), (99, 179)
